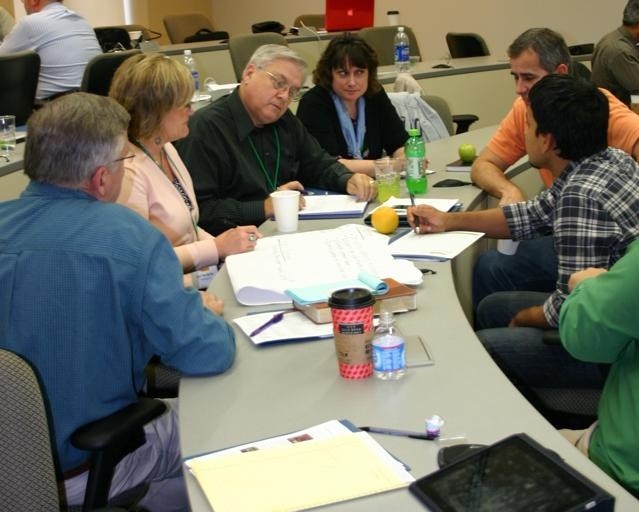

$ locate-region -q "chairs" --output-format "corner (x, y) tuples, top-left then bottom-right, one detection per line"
(1, 348), (166, 512)
(0, 6), (598, 204)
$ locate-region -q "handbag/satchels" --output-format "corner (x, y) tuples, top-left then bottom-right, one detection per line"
(184, 28), (229, 42)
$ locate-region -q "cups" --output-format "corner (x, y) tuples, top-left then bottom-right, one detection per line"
(374, 157), (402, 204)
(329, 288), (375, 379)
(129, 31), (144, 49)
(410, 56), (419, 72)
(387, 11), (400, 26)
(0, 114), (17, 162)
(270, 190), (299, 232)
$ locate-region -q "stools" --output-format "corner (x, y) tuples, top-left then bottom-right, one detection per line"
(139, 357), (179, 400)
(526, 384), (602, 430)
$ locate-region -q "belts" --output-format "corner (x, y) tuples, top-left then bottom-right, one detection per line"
(36, 88), (81, 111)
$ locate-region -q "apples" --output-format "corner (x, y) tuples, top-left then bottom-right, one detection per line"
(458, 143), (475, 163)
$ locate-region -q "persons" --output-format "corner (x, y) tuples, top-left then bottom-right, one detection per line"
(176, 54), (376, 236)
(0, 89), (237, 507)
(556, 239), (639, 497)
(591, 0), (639, 110)
(405, 73), (639, 389)
(470, 27), (639, 311)
(296, 30), (430, 178)
(0, 0), (104, 110)
(107, 50), (262, 287)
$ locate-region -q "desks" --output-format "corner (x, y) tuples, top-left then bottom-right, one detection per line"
(176, 95), (639, 512)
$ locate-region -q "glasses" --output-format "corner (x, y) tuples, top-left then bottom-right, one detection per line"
(106, 147), (135, 168)
(259, 68), (304, 102)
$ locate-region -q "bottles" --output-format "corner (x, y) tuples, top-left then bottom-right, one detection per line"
(370, 311), (405, 380)
(393, 26), (411, 73)
(405, 130), (428, 192)
(181, 50), (200, 111)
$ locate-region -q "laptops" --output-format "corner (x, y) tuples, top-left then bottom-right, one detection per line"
(316, 0), (374, 32)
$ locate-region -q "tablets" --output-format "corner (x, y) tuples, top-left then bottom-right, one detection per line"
(408, 433), (615, 512)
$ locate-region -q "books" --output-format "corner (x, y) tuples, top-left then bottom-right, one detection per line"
(292, 277), (418, 325)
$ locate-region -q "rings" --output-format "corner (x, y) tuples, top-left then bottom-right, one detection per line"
(369, 180), (374, 184)
(247, 234), (256, 241)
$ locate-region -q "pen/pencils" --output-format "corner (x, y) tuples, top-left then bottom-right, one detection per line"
(410, 193), (420, 235)
(250, 314), (283, 337)
(358, 427), (434, 440)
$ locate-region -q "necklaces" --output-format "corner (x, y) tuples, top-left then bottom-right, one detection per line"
(158, 151), (163, 169)
